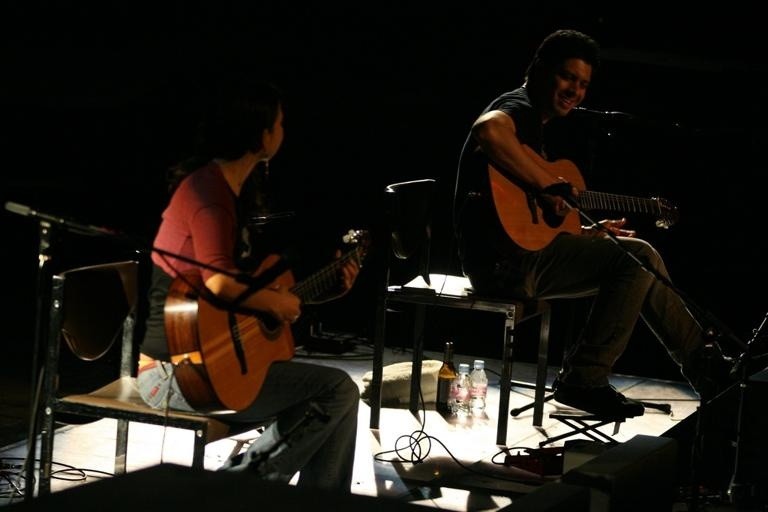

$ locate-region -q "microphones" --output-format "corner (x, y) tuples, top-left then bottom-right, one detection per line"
(570, 106), (632, 125)
(228, 245), (306, 310)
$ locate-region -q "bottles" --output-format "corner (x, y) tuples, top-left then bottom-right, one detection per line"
(436, 340), (458, 412)
(447, 359), (490, 418)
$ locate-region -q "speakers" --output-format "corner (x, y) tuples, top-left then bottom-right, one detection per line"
(496, 435), (678, 512)
(659, 372), (768, 437)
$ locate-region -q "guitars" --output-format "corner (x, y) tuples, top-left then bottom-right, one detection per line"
(488, 144), (680, 253)
(163, 230), (370, 411)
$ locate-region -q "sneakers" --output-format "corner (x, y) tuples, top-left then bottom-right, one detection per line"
(551, 368), (644, 417)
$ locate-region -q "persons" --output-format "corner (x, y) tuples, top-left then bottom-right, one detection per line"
(452, 25), (766, 416)
(134, 84), (359, 512)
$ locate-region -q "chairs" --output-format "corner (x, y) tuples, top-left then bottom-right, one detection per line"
(39, 260), (301, 512)
(369, 175), (557, 446)
